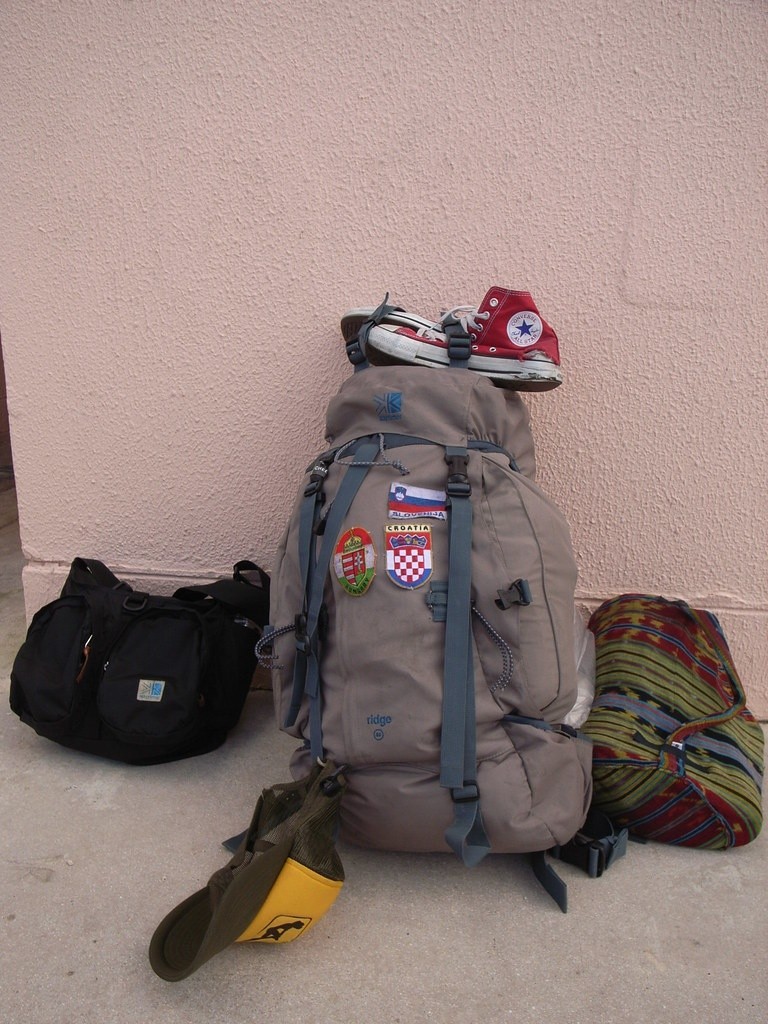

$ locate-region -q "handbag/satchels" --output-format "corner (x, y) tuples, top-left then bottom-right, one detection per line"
(10, 555), (253, 766)
(172, 561), (269, 626)
(587, 594), (768, 851)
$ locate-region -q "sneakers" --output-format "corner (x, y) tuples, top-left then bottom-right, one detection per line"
(366, 285), (563, 392)
(340, 306), (448, 366)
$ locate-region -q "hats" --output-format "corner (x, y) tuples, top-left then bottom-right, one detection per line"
(147, 763), (350, 983)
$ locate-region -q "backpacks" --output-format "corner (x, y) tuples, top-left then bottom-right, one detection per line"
(254, 368), (628, 913)
